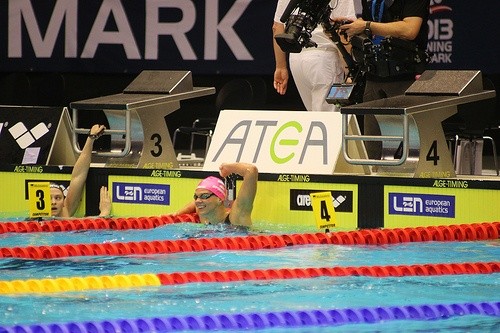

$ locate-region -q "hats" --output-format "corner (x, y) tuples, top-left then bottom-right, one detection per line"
(49, 181), (63, 191)
(196, 175), (226, 202)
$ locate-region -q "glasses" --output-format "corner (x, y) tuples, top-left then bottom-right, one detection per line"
(194, 193), (215, 200)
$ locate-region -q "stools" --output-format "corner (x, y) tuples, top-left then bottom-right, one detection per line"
(172, 77), (267, 157)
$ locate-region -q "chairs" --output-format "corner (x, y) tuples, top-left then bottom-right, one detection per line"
(443, 77), (500, 176)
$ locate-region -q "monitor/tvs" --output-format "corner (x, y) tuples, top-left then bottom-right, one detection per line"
(326, 83), (357, 105)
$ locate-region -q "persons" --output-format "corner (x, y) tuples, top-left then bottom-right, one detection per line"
(340, 0), (428, 159)
(271, 0), (357, 111)
(171, 162), (257, 230)
(50, 125), (111, 218)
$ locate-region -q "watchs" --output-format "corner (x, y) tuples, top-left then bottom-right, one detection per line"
(365, 21), (371, 37)
